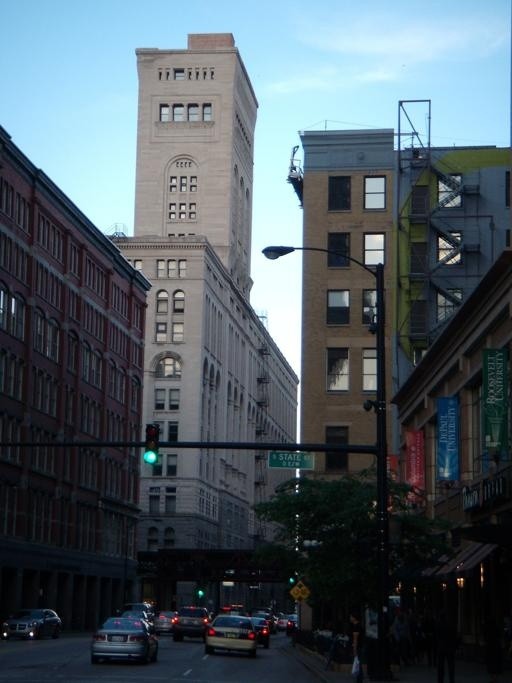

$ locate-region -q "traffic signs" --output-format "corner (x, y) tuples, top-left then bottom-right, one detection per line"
(268, 451), (314, 468)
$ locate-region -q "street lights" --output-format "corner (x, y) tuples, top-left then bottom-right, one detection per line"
(262, 242), (390, 683)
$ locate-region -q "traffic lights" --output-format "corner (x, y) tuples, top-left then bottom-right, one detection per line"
(289, 575), (297, 587)
(143, 423), (158, 463)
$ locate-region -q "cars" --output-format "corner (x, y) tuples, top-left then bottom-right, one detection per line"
(89, 602), (300, 664)
(1, 608), (62, 638)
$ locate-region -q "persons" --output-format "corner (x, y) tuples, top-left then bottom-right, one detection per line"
(337, 604), (512, 682)
